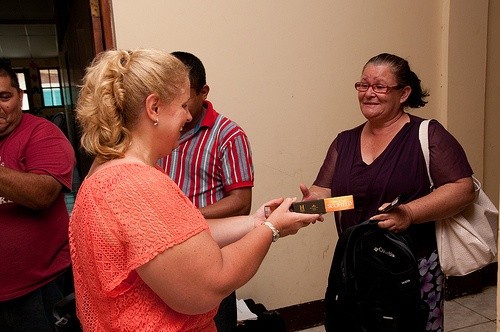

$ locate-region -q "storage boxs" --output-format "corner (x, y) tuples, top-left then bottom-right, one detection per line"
(264, 194), (355, 219)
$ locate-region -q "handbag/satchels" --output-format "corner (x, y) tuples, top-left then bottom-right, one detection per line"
(419, 119), (500, 276)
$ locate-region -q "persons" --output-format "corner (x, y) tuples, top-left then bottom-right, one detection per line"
(299, 53), (475, 332)
(156, 51), (254, 332)
(0, 63), (76, 332)
(68, 48), (322, 332)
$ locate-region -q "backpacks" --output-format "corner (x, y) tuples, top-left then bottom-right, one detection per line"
(323, 221), (428, 332)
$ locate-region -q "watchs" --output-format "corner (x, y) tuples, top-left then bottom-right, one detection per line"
(262, 221), (280, 242)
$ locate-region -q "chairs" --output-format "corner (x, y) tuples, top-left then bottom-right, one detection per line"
(51, 112), (65, 128)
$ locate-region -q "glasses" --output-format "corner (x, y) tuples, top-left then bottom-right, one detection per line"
(355, 82), (401, 93)
